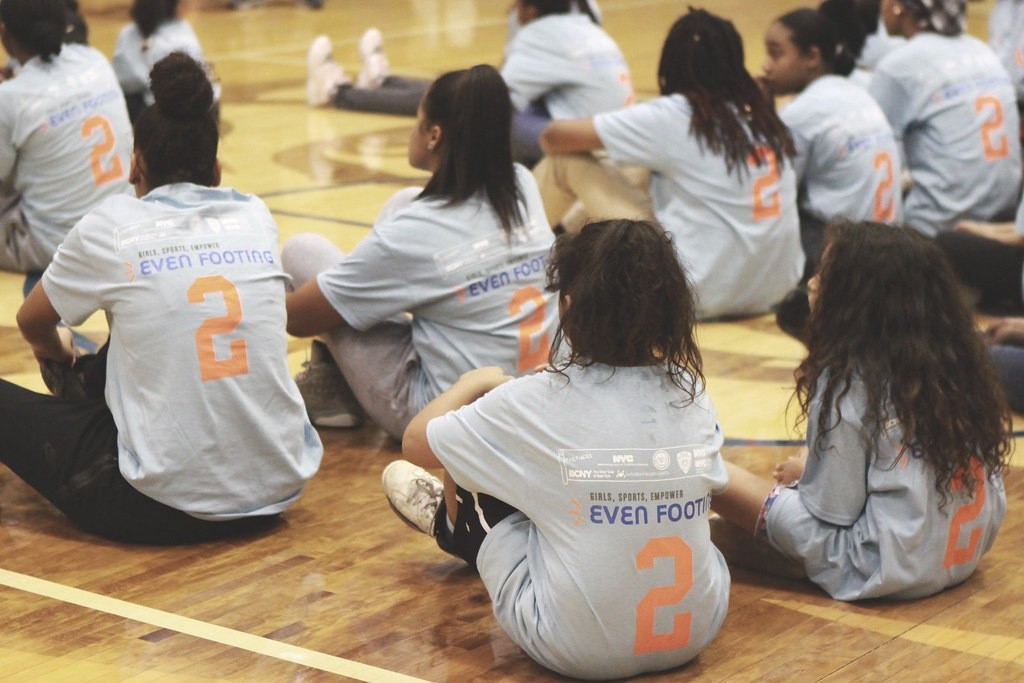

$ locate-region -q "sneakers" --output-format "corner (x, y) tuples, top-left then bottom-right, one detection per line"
(355, 28), (388, 89)
(305, 36), (352, 107)
(293, 365), (362, 428)
(381, 459), (444, 538)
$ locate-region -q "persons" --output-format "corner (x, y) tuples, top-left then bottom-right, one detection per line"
(0, 0), (1023, 444)
(381, 219), (732, 683)
(709, 212), (1017, 600)
(0, 51), (323, 547)
